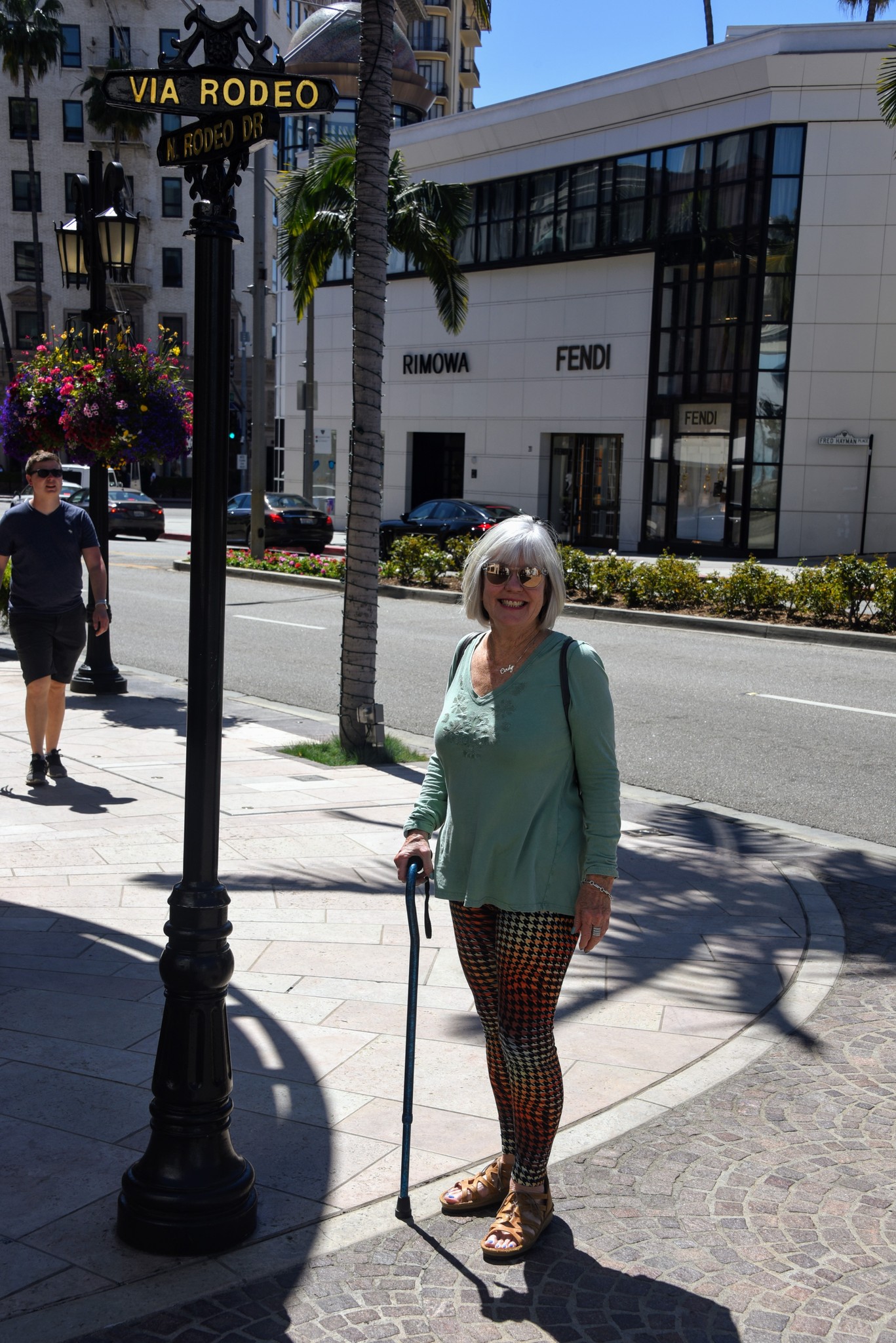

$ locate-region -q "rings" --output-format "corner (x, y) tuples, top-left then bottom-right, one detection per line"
(592, 928), (601, 936)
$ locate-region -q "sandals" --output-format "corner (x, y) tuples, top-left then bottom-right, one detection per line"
(482, 1177), (554, 1256)
(440, 1154), (518, 1209)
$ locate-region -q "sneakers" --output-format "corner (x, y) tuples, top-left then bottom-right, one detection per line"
(26, 753), (48, 784)
(45, 749), (67, 778)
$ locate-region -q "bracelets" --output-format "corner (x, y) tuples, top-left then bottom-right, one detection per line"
(581, 877), (612, 900)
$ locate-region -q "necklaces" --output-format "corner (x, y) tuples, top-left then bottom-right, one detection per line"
(490, 632), (539, 674)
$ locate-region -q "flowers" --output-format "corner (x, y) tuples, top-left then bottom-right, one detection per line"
(0, 317), (196, 470)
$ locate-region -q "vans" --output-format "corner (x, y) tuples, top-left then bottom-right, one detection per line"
(60, 463), (124, 488)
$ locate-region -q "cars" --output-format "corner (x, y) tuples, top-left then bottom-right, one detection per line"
(61, 485), (165, 542)
(377, 499), (526, 567)
(676, 502), (727, 541)
(226, 491), (334, 555)
(10, 479), (89, 509)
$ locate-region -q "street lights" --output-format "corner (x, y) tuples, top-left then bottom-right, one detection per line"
(52, 147), (254, 294)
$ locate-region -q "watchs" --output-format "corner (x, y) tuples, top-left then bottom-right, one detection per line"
(95, 599), (109, 609)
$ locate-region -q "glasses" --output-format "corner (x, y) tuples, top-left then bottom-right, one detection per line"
(482, 563), (547, 589)
(31, 468), (64, 478)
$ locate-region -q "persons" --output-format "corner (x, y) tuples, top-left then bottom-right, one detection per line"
(394, 514), (621, 1257)
(0, 450), (109, 783)
